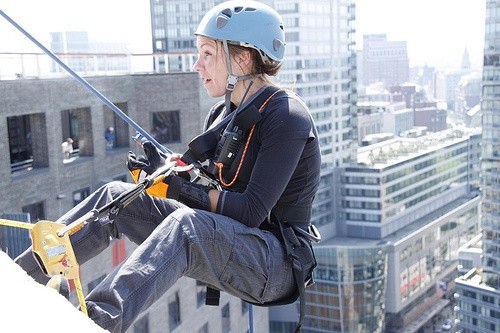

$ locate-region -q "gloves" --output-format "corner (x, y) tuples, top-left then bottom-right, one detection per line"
(126, 140), (185, 200)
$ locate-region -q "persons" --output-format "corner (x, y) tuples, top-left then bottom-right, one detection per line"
(61, 138), (74, 154)
(15, 0), (321, 333)
(105, 127), (115, 144)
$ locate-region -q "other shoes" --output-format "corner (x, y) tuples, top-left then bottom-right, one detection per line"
(47, 274), (70, 299)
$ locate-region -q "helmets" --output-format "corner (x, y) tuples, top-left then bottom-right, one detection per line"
(196, 0), (285, 62)
